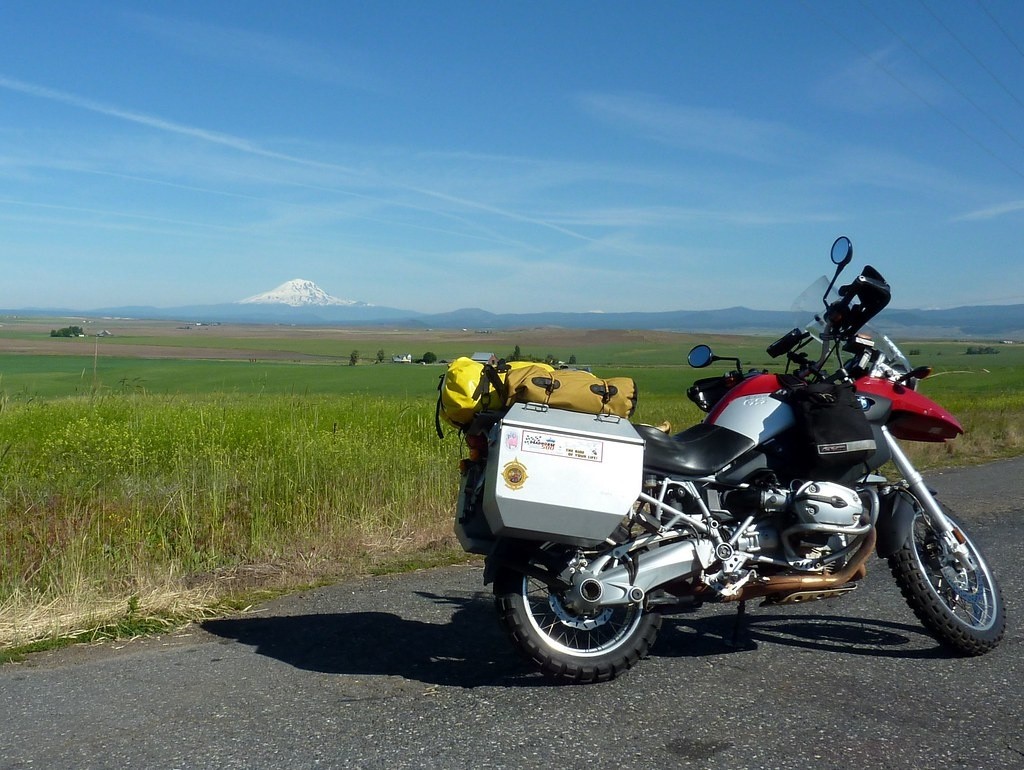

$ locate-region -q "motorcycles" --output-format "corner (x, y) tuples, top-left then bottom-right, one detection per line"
(454, 231), (1008, 685)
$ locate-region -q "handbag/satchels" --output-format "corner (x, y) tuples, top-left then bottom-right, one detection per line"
(434, 356), (640, 439)
